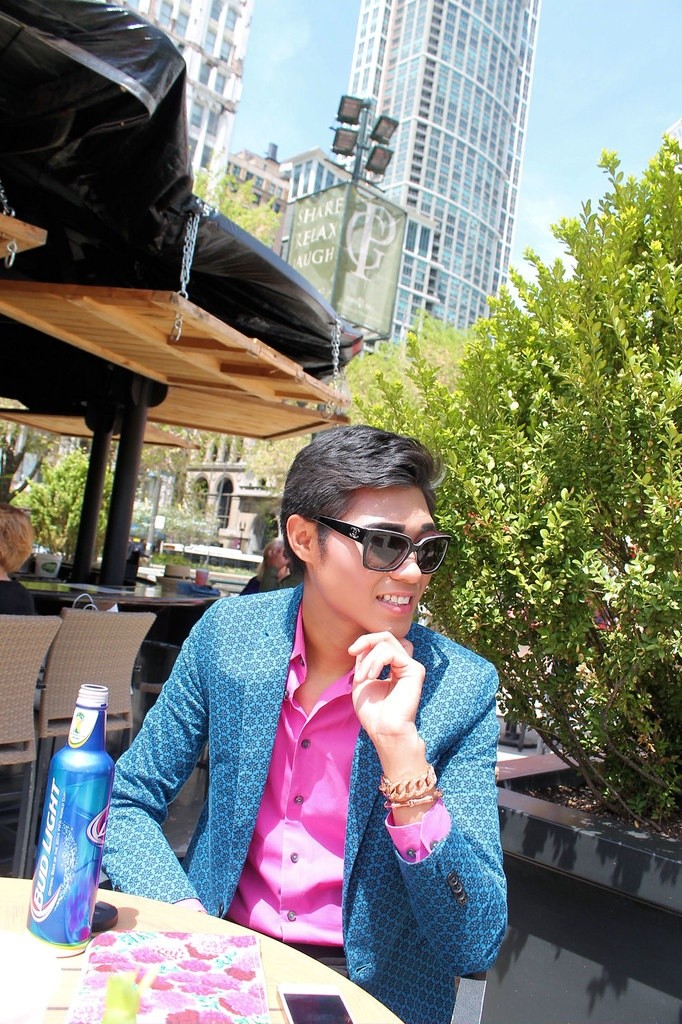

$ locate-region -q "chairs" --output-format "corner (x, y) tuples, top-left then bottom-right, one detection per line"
(445, 970), (488, 1024)
(1, 615), (62, 878)
(137, 682), (209, 802)
(24, 606), (156, 880)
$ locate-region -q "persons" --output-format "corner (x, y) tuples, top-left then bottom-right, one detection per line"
(237, 538), (289, 595)
(97, 424), (509, 1024)
(259, 544), (304, 593)
(0, 504), (35, 616)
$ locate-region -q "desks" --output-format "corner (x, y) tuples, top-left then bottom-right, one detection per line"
(19, 560), (230, 606)
(0, 877), (403, 1024)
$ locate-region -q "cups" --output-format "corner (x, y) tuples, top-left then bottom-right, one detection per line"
(195, 568), (208, 587)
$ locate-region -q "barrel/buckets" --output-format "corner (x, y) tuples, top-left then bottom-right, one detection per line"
(34, 553), (63, 578)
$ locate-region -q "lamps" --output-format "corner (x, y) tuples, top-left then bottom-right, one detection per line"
(332, 95), (399, 176)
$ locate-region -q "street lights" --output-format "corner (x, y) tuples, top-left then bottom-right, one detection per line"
(330, 92), (400, 311)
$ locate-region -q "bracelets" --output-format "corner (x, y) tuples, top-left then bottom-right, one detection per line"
(383, 788), (443, 809)
(377, 761), (437, 801)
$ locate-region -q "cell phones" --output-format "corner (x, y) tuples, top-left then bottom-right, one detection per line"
(276, 986), (355, 1023)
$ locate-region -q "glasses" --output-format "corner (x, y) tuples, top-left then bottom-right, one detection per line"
(307, 512), (453, 578)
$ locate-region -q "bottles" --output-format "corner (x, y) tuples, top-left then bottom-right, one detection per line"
(22, 683), (117, 960)
